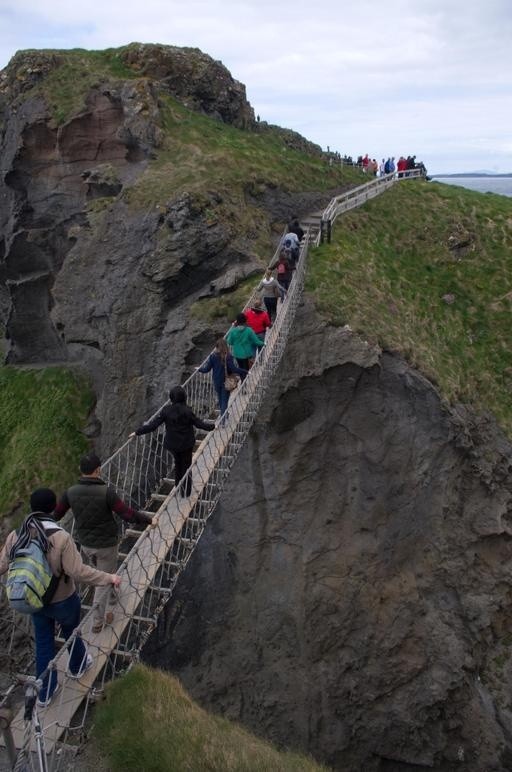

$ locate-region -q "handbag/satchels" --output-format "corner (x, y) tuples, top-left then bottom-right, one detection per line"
(225, 376), (237, 391)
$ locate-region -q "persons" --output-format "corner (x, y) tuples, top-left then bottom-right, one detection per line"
(255, 269), (288, 327)
(243, 299), (275, 366)
(52, 455), (159, 634)
(128, 385), (219, 498)
(0, 488), (122, 707)
(335, 151), (426, 181)
(267, 220), (306, 303)
(225, 313), (267, 394)
(195, 339), (252, 429)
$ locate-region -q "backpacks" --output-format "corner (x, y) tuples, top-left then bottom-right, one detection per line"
(6, 528), (62, 614)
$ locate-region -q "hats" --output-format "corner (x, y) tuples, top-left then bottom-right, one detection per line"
(31, 488), (56, 512)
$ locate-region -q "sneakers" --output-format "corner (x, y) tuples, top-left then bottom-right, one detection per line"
(38, 684), (59, 707)
(92, 613), (113, 633)
(70, 654), (93, 679)
